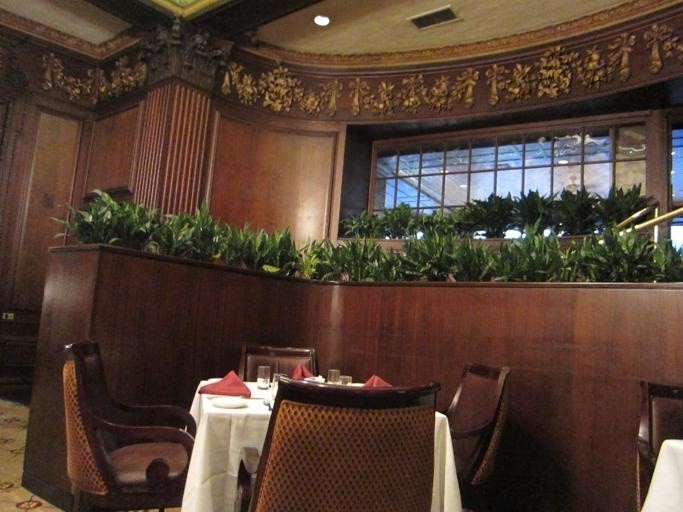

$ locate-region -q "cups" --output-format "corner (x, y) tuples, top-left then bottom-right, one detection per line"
(327, 369), (353, 387)
(256, 365), (270, 389)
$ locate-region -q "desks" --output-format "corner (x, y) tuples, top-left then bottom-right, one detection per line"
(640, 438), (682, 512)
(182, 377), (464, 511)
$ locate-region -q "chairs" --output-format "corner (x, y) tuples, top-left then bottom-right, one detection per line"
(445, 363), (513, 512)
(635, 377), (683, 512)
(238, 341), (319, 383)
(65, 353), (197, 512)
(234, 376), (442, 512)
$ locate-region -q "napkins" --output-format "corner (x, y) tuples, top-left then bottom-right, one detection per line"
(198, 370), (250, 397)
(363, 376), (391, 389)
(293, 364), (314, 380)
